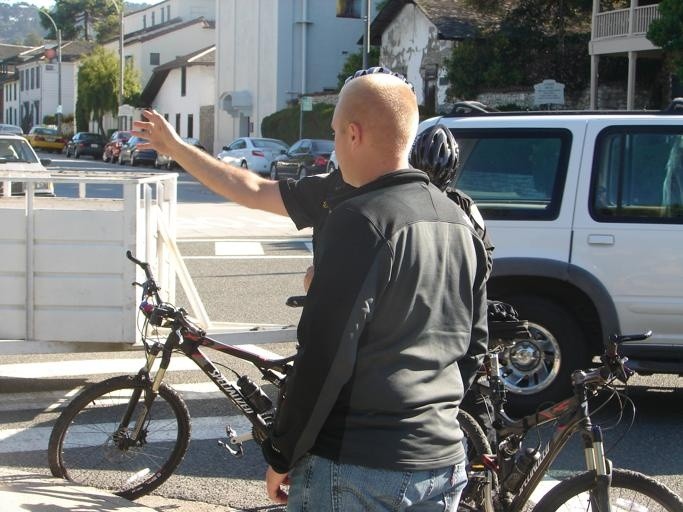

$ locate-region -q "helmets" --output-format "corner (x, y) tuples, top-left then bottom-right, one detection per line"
(344, 67), (415, 95)
(408, 124), (460, 191)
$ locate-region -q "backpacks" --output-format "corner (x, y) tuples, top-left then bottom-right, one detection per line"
(486, 299), (520, 321)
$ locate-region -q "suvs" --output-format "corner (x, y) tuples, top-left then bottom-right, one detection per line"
(408, 96), (683, 420)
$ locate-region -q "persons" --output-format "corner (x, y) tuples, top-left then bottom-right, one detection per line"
(660, 96), (683, 218)
(260, 73), (488, 512)
(130, 65), (447, 293)
(303, 117), (494, 293)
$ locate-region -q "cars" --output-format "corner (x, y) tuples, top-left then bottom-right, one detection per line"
(26, 127), (64, 153)
(0, 123), (23, 135)
(214, 137), (289, 174)
(153, 137), (206, 169)
(65, 132), (110, 159)
(118, 135), (155, 166)
(326, 150), (338, 174)
(0, 135), (55, 198)
(100, 130), (140, 163)
(268, 139), (334, 182)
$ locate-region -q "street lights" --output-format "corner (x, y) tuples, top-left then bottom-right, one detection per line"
(18, 3), (62, 134)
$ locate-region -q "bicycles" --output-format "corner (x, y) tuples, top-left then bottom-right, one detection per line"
(47, 250), (491, 503)
(457, 328), (683, 512)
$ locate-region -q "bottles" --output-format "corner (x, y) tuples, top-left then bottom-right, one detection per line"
(502, 447), (541, 494)
(236, 374), (273, 415)
